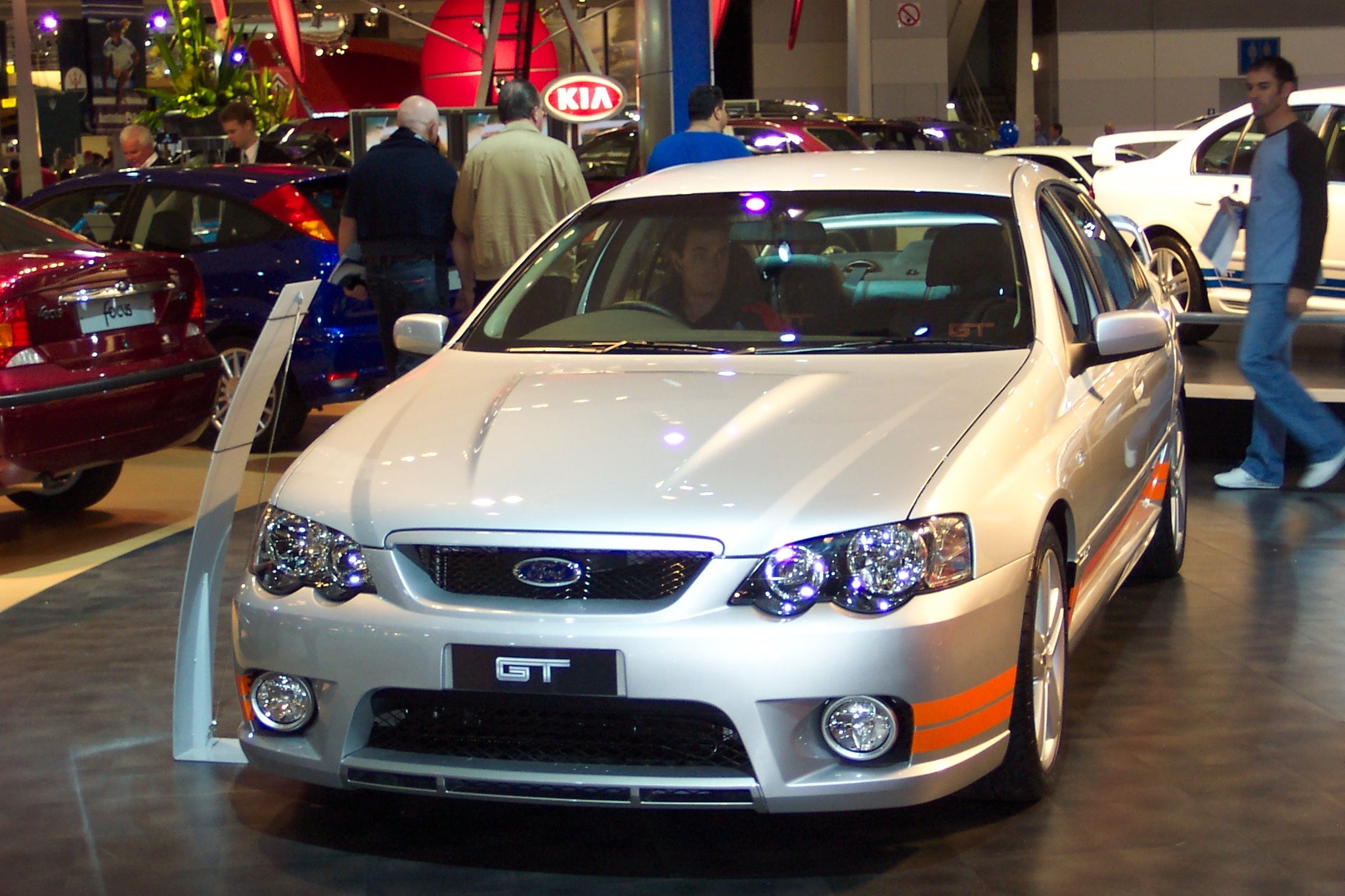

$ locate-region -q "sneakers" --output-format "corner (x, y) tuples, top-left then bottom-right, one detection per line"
(1297, 447), (1345, 488)
(1213, 467), (1279, 489)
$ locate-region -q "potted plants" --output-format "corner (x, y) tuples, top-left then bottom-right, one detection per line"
(131, 0), (258, 164)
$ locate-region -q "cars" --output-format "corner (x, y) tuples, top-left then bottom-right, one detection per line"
(0, 199), (224, 516)
(14, 160), (463, 453)
(170, 148), (1190, 815)
(572, 96), (1267, 256)
(1090, 82), (1345, 348)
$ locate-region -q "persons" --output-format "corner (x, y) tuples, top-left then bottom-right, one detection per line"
(453, 79), (591, 349)
(338, 95), (474, 387)
(119, 125), (179, 225)
(644, 85), (752, 177)
(104, 18), (139, 116)
(220, 104), (293, 226)
(997, 114), (1120, 157)
(641, 216), (769, 330)
(0, 143), (115, 203)
(1214, 54), (1345, 488)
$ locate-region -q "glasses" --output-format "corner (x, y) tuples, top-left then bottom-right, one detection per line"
(529, 105), (548, 117)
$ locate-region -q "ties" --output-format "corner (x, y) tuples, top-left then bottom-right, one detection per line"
(241, 153), (249, 164)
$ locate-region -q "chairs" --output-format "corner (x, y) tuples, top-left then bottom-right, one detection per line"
(908, 221), (1026, 343)
(753, 254), (854, 335)
